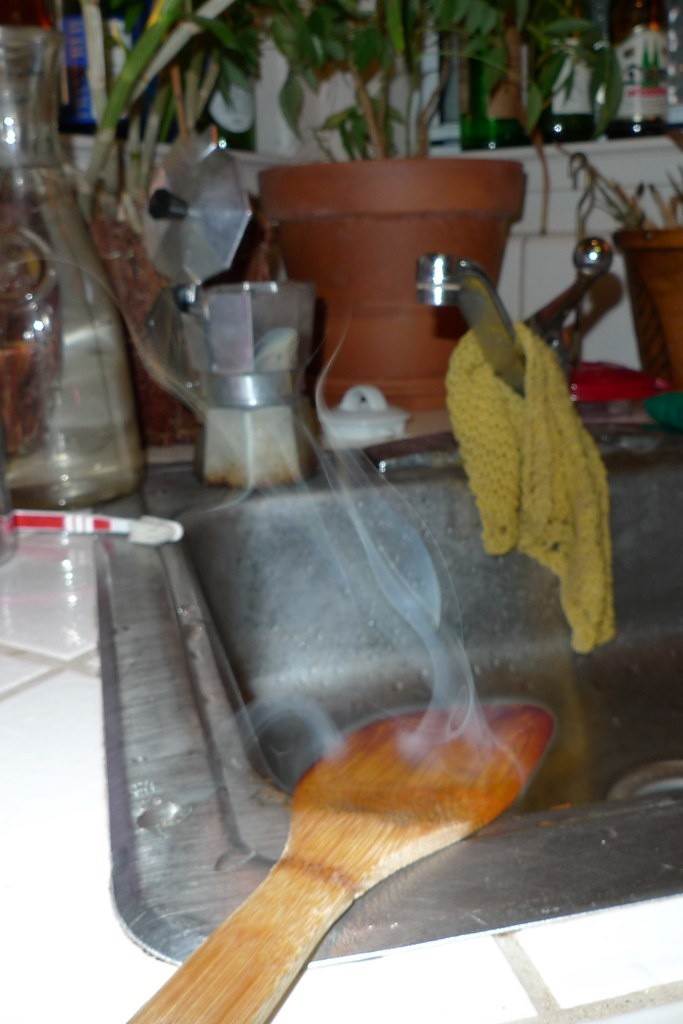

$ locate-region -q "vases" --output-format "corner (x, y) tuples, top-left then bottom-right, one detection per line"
(610, 225), (683, 396)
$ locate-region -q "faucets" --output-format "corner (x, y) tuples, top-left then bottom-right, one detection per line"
(413, 234), (615, 402)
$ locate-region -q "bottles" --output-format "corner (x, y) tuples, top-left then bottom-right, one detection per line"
(607, 0), (668, 136)
(458, 0), (524, 153)
(535, 0), (594, 142)
(1, 25), (143, 512)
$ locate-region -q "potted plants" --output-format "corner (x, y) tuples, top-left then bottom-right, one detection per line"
(37, 1), (626, 443)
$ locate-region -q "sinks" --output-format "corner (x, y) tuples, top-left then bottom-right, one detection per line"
(91, 419), (681, 969)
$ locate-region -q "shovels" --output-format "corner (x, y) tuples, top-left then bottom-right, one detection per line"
(126, 702), (557, 1024)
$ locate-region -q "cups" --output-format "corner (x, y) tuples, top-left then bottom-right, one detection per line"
(136, 132), (317, 492)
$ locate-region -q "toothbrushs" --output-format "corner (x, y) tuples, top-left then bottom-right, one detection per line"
(0, 506), (187, 546)
